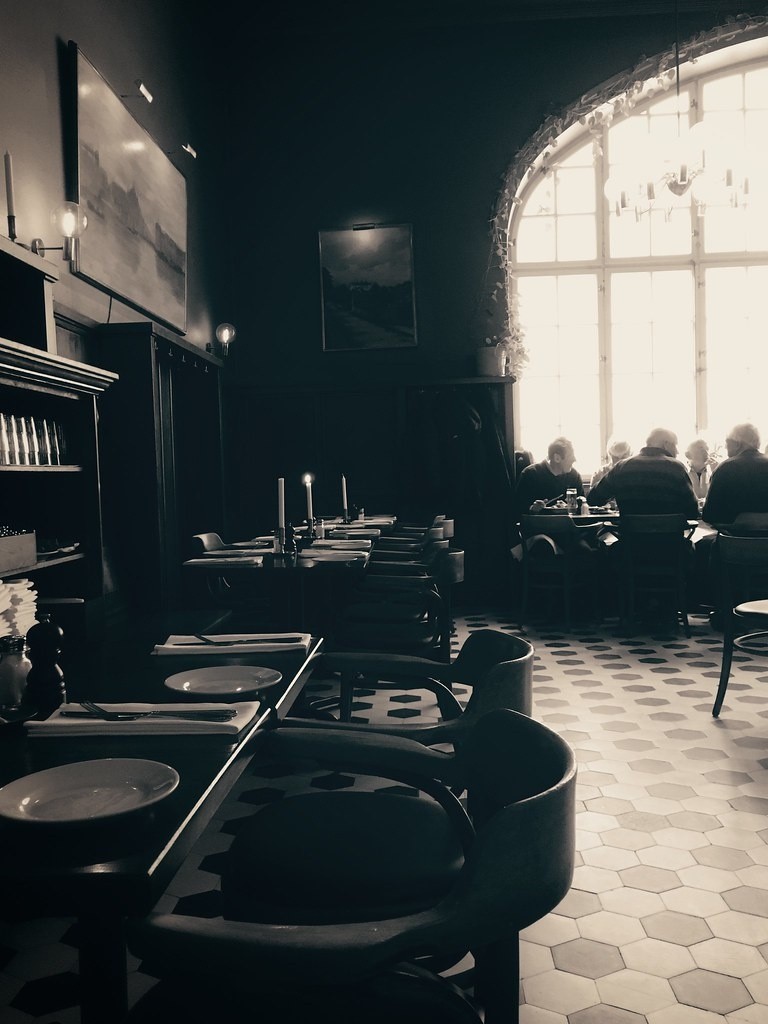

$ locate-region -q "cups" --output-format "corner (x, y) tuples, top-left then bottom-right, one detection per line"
(566, 487), (577, 515)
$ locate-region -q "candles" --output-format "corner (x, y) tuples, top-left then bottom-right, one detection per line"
(3, 150), (15, 215)
(278, 478), (285, 527)
(303, 474), (312, 519)
(342, 475), (347, 510)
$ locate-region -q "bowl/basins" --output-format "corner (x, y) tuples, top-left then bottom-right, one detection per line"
(0, 703), (39, 731)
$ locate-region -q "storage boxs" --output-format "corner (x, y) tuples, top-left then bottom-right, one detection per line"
(0, 533), (38, 573)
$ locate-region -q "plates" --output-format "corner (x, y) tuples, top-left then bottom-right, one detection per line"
(164, 666), (284, 695)
(224, 514), (395, 562)
(541, 506), (576, 515)
(0, 758), (180, 823)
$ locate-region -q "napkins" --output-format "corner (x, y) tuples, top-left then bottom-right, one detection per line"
(203, 547), (274, 554)
(300, 547), (368, 557)
(251, 535), (302, 541)
(310, 539), (372, 548)
(329, 529), (381, 535)
(149, 632), (312, 654)
(183, 555), (264, 566)
(22, 701), (261, 737)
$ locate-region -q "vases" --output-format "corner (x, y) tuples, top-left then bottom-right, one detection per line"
(475, 347), (507, 376)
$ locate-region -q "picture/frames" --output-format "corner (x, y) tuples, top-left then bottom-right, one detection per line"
(68, 39), (189, 335)
(318, 221), (418, 352)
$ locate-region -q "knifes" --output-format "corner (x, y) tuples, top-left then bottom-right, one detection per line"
(173, 637), (302, 645)
(60, 709), (233, 722)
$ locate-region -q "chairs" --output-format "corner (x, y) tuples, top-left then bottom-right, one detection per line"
(179, 532), (275, 612)
(130, 627), (574, 1024)
(519, 514), (768, 717)
(335, 515), (465, 720)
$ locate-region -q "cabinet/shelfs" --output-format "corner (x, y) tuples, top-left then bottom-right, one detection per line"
(0, 233), (120, 605)
(224, 375), (516, 537)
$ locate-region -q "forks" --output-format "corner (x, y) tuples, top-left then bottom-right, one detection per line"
(81, 701), (238, 721)
(195, 633), (302, 646)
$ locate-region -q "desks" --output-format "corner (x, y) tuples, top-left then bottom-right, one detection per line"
(521, 512), (620, 526)
(190, 513), (398, 719)
(0, 635), (325, 1024)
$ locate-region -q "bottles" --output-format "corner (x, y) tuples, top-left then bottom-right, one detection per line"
(0, 606), (67, 708)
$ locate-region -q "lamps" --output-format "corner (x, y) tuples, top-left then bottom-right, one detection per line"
(205, 323), (236, 356)
(32, 201), (87, 261)
(120, 79), (153, 104)
(168, 143), (196, 158)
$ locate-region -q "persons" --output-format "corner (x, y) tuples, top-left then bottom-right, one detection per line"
(590, 438), (768, 511)
(514, 437), (592, 554)
(702, 423), (768, 618)
(587, 428), (700, 621)
